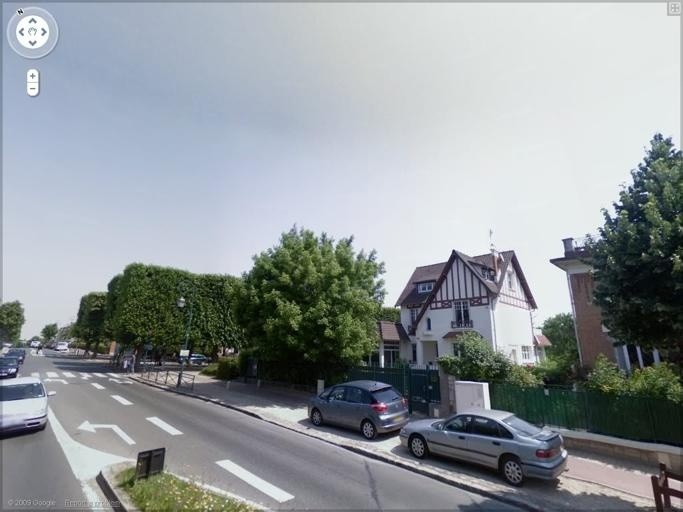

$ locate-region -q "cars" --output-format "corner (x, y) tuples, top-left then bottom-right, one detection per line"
(178, 353), (207, 365)
(0, 343), (26, 377)
(396, 406), (568, 484)
(29, 340), (70, 352)
(0, 378), (56, 438)
(305, 380), (408, 439)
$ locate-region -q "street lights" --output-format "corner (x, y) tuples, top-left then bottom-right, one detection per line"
(175, 287), (196, 389)
